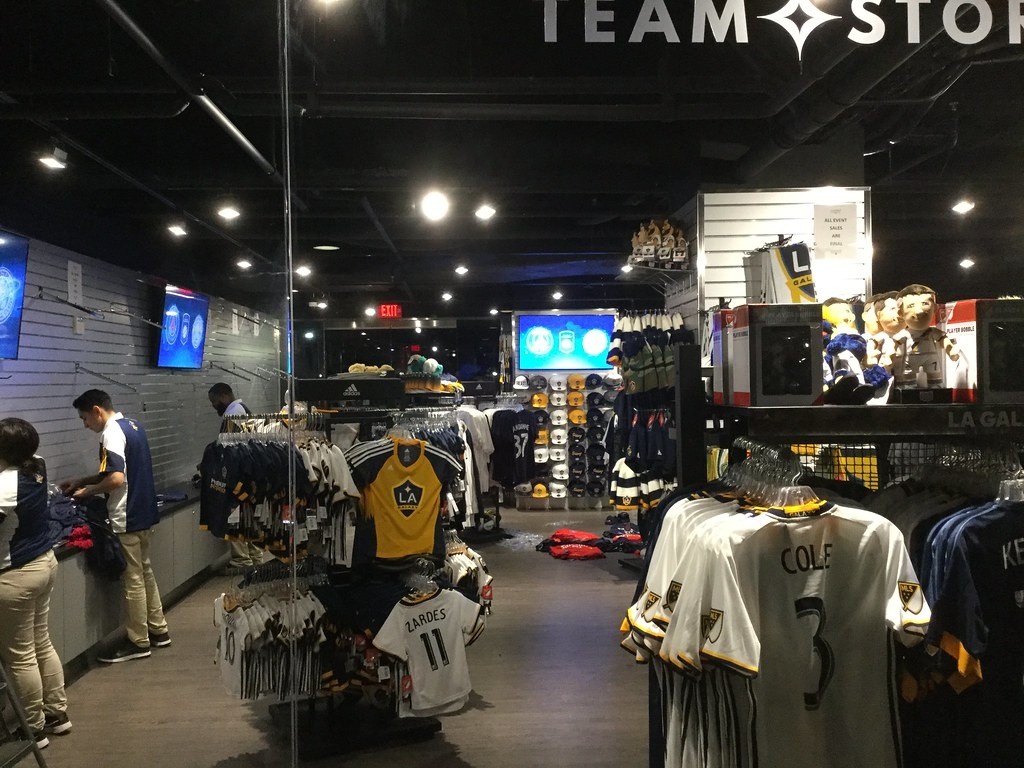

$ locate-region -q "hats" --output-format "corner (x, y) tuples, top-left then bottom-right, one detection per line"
(512, 373), (622, 498)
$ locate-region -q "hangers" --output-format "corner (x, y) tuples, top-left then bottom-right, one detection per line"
(703, 433), (1024, 519)
(621, 308), (683, 412)
(215, 392), (524, 613)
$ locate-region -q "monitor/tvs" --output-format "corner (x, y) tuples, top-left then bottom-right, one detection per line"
(518, 315), (615, 371)
(0, 227), (30, 360)
(155, 280), (210, 369)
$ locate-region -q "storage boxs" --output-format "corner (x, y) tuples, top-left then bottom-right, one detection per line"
(732, 301), (824, 406)
(945, 297), (1023, 402)
(711, 308), (732, 404)
(930, 303), (946, 368)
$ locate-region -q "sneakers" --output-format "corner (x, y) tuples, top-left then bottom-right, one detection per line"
(96, 640), (151, 664)
(149, 632), (171, 647)
(43, 712), (71, 734)
(14, 728), (49, 749)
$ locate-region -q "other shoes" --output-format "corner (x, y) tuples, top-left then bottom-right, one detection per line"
(217, 565), (249, 576)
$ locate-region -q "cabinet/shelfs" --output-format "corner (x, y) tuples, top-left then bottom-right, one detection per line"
(455, 380), (528, 544)
(220, 377), (459, 759)
(650, 343), (1024, 768)
(47, 481), (232, 664)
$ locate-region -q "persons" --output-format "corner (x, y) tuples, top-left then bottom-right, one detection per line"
(0, 417), (74, 750)
(58, 389), (172, 663)
(819, 284), (960, 406)
(208, 383), (268, 575)
